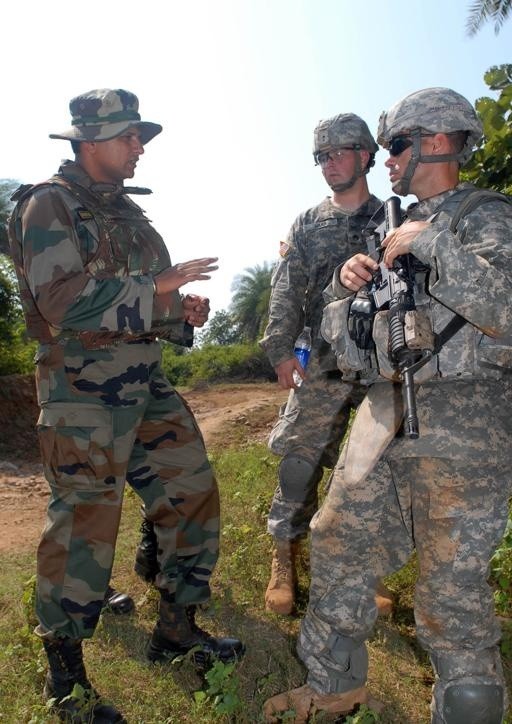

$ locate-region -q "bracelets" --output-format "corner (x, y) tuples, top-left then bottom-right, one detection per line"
(148, 272), (157, 298)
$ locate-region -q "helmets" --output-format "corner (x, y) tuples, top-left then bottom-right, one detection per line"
(313, 112), (379, 154)
(377, 86), (483, 149)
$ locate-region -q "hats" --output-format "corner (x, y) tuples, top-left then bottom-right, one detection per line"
(50, 87), (163, 146)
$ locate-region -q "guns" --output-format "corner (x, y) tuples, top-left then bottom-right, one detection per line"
(362, 196), (434, 440)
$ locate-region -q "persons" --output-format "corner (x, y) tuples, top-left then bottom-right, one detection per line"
(262, 111), (399, 615)
(257, 84), (511, 721)
(9, 89), (242, 722)
(103, 503), (161, 613)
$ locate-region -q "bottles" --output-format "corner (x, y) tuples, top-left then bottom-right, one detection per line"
(290, 326), (313, 387)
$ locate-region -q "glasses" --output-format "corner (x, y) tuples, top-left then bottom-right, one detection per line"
(316, 149), (343, 167)
(389, 136), (413, 156)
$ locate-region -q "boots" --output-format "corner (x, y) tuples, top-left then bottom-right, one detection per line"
(101, 584), (135, 616)
(146, 593), (246, 672)
(374, 577), (396, 618)
(134, 515), (158, 581)
(43, 637), (127, 723)
(262, 682), (368, 723)
(264, 535), (299, 616)
